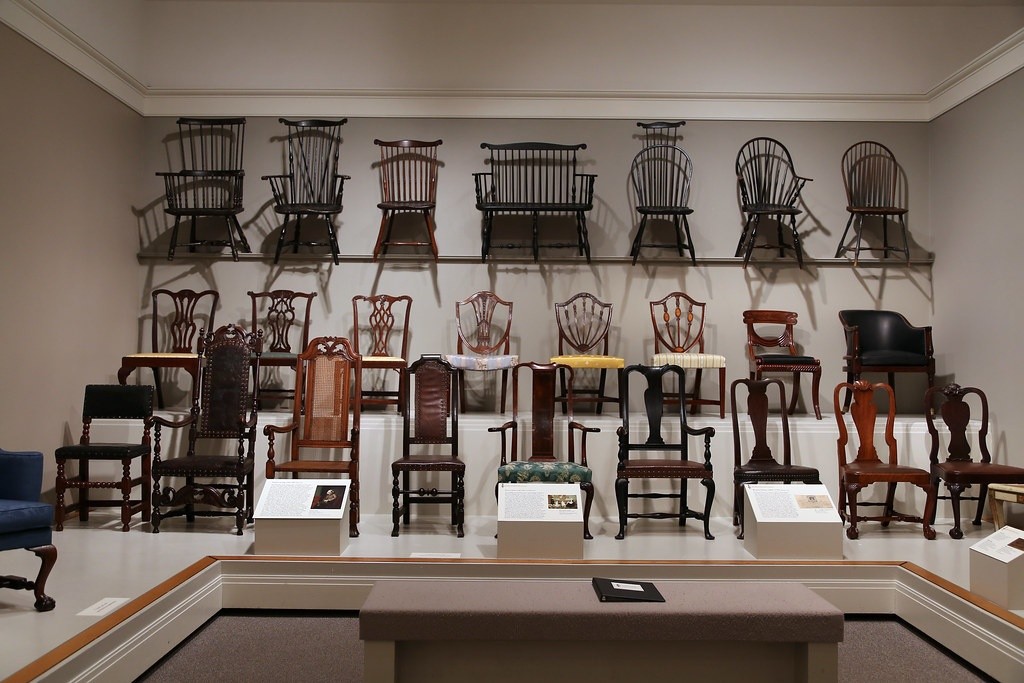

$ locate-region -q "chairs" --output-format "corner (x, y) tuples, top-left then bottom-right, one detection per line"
(350, 295), (412, 416)
(262, 119), (351, 264)
(263, 337), (363, 538)
(146, 324), (263, 535)
(988, 483), (1024, 532)
(924, 383), (1024, 539)
(0, 449), (57, 612)
(392, 355), (465, 537)
(731, 378), (821, 539)
(117, 289), (218, 407)
(372, 138), (443, 265)
(742, 310), (823, 421)
(834, 380), (936, 540)
(650, 292), (726, 419)
(835, 141), (912, 269)
(839, 309), (936, 418)
(629, 121), (697, 266)
(55, 385), (153, 531)
(488, 361), (601, 539)
(551, 292), (625, 418)
(247, 290), (318, 415)
(614, 364), (715, 539)
(447, 291), (518, 414)
(735, 138), (813, 268)
(157, 114), (250, 260)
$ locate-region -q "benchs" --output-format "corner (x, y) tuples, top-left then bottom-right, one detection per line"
(358, 576), (845, 683)
(472, 142), (597, 264)
